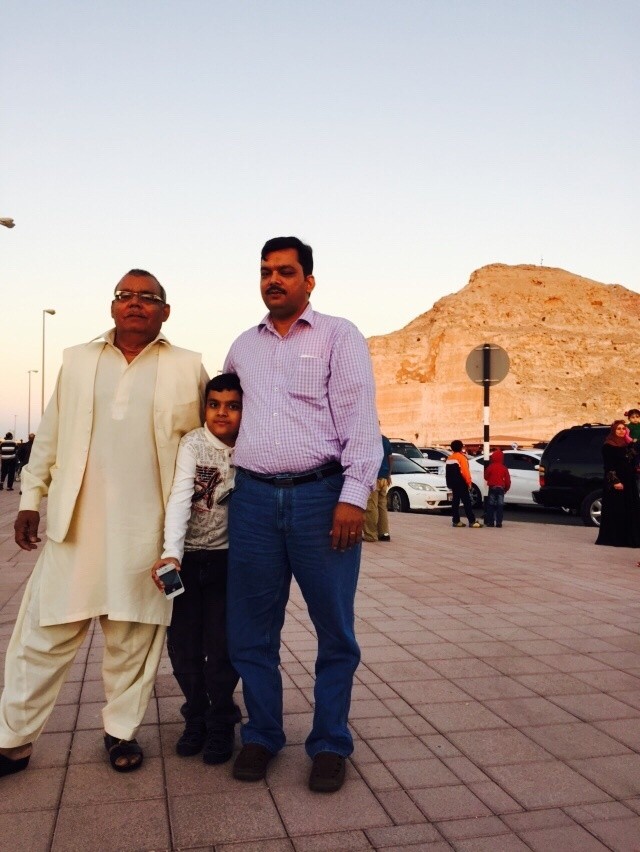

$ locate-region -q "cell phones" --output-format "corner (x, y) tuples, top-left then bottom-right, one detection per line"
(156, 563), (184, 600)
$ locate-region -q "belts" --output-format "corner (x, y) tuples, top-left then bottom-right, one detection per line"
(240, 463), (342, 485)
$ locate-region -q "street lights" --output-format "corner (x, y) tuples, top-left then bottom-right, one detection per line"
(27, 370), (39, 438)
(42, 309), (56, 418)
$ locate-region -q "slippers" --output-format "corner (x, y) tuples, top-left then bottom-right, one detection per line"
(0, 756), (30, 779)
(104, 733), (142, 771)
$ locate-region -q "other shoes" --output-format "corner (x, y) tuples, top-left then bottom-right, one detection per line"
(469, 521), (481, 528)
(379, 534), (391, 541)
(309, 752), (346, 791)
(235, 743), (272, 780)
(176, 726), (233, 763)
(454, 522), (465, 527)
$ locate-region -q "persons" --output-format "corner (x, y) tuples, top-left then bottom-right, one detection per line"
(0, 433), (36, 495)
(222, 237), (385, 792)
(480, 448), (512, 529)
(592, 408), (640, 549)
(446, 440), (484, 529)
(151, 372), (246, 767)
(363, 419), (393, 543)
(0, 266), (210, 779)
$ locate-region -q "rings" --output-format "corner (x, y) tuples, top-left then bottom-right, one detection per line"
(350, 532), (356, 534)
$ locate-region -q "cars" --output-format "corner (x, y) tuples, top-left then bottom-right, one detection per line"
(384, 453), (464, 515)
(417, 447), (452, 462)
(467, 448), (544, 513)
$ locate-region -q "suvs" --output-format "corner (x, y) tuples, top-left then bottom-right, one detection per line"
(387, 436), (445, 476)
(531, 423), (612, 528)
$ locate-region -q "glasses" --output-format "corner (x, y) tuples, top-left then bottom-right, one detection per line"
(115, 289), (164, 302)
